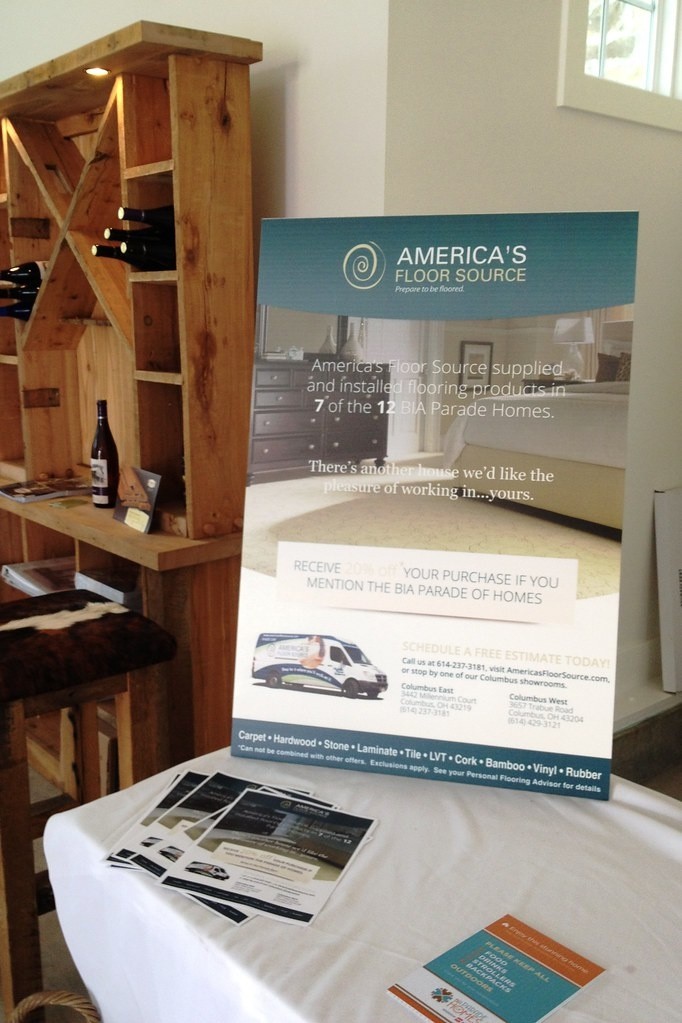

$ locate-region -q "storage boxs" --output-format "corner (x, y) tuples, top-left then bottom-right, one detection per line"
(155, 502), (189, 538)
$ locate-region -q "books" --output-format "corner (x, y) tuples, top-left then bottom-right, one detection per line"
(0, 476), (94, 503)
(74, 567), (141, 799)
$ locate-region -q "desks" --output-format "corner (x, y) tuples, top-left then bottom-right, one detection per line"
(43, 745), (682, 1023)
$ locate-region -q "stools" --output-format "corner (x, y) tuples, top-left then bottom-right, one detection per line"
(0, 588), (181, 1023)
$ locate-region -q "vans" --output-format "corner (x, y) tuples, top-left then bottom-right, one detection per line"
(142, 835), (162, 849)
(184, 860), (230, 881)
(160, 845), (184, 863)
(251, 630), (388, 700)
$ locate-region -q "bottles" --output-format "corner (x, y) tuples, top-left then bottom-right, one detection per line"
(91, 400), (119, 508)
(118, 206), (174, 226)
(91, 228), (175, 270)
(0, 261), (50, 321)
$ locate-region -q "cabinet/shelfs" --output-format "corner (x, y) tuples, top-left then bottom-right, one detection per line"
(0, 20), (266, 801)
